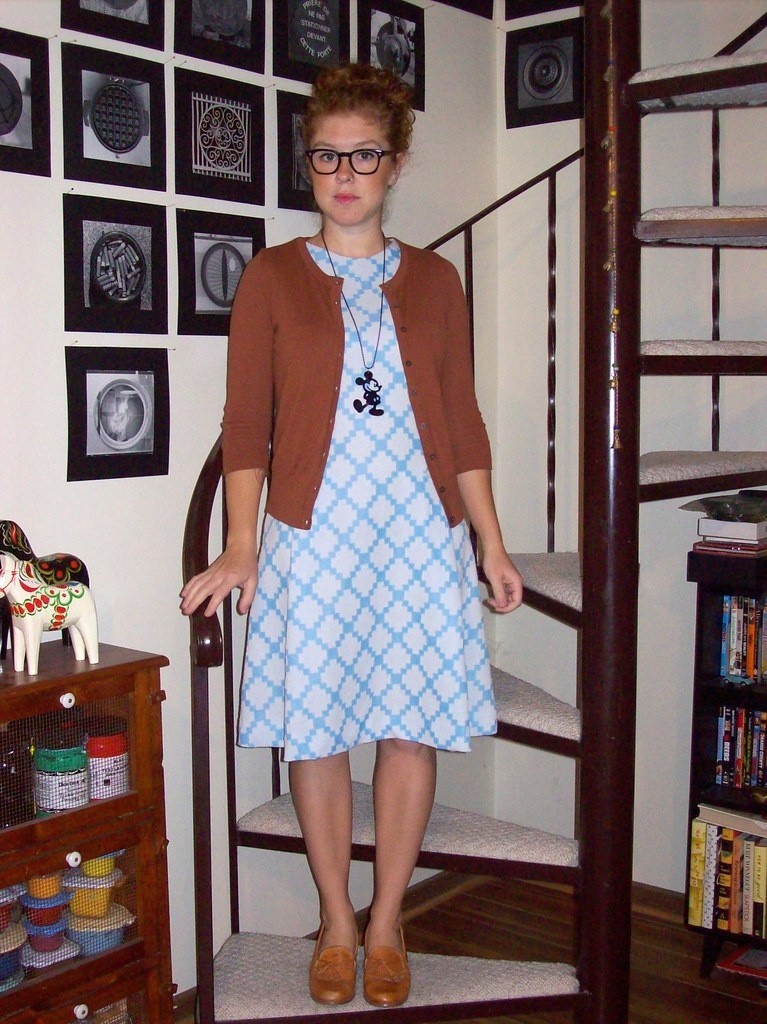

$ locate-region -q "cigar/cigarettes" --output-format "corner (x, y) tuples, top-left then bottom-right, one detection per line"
(96, 240), (140, 297)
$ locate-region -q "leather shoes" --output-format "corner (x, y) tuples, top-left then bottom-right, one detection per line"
(309, 921), (359, 1004)
(364, 922), (410, 1007)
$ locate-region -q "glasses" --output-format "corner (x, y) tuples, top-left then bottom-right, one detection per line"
(301, 144), (393, 175)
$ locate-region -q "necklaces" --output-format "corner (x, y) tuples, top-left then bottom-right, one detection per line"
(319, 231), (385, 416)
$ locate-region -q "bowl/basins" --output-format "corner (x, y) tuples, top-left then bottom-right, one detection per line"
(0, 849), (138, 992)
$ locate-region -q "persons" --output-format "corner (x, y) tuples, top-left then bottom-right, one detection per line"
(180, 65), (524, 1003)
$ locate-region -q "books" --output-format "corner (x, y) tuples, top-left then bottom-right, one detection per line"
(688, 496), (766, 937)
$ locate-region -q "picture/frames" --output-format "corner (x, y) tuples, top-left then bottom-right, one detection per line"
(0, 0), (583, 337)
(64, 346), (170, 482)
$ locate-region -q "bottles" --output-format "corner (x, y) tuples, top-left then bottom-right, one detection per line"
(0, 707), (131, 828)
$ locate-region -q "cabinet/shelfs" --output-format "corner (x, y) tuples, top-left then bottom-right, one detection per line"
(685, 552), (767, 987)
(0, 639), (178, 1024)
